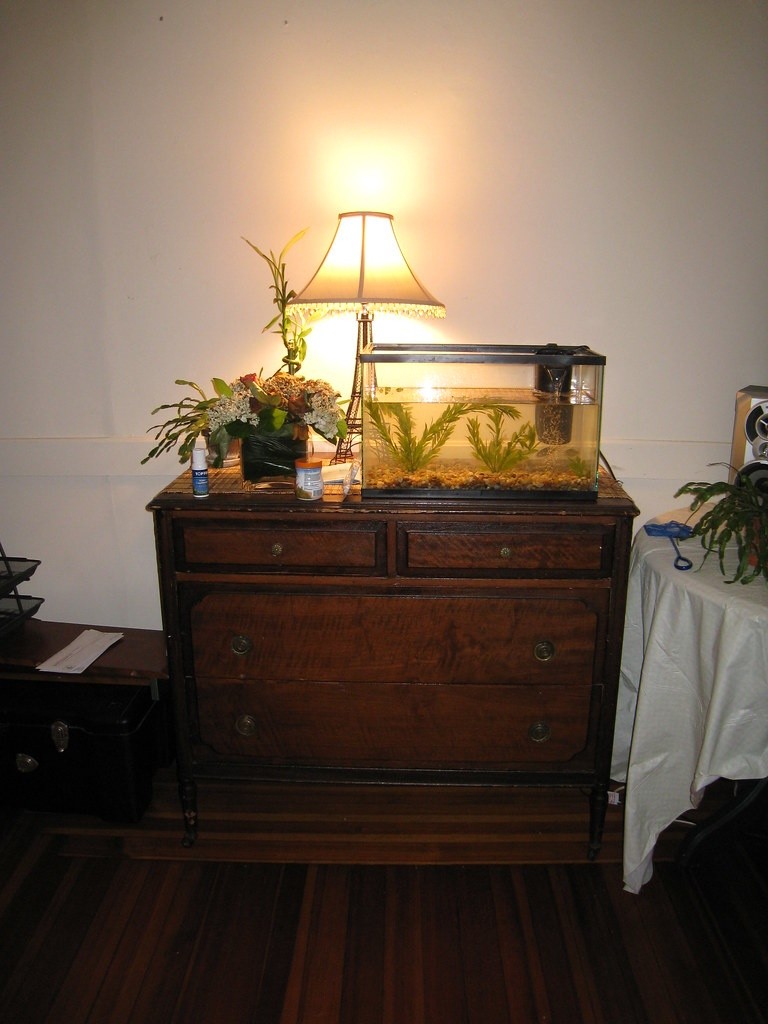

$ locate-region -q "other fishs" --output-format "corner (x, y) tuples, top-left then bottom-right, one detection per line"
(537, 446), (580, 458)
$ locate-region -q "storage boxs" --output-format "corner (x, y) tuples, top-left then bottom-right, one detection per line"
(4, 679), (176, 823)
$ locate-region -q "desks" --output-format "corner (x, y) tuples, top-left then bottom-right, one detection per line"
(610, 503), (768, 895)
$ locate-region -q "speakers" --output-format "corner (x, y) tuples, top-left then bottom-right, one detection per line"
(727, 385), (768, 511)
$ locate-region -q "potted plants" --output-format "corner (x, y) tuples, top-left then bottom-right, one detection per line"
(673, 462), (768, 585)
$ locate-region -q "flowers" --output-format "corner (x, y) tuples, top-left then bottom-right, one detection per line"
(141, 227), (352, 468)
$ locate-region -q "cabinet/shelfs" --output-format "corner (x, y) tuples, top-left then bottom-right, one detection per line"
(146, 454), (641, 860)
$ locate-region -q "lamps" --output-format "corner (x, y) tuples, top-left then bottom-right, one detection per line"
(286, 212), (446, 466)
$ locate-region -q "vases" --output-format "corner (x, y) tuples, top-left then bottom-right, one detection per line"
(240, 433), (308, 485)
